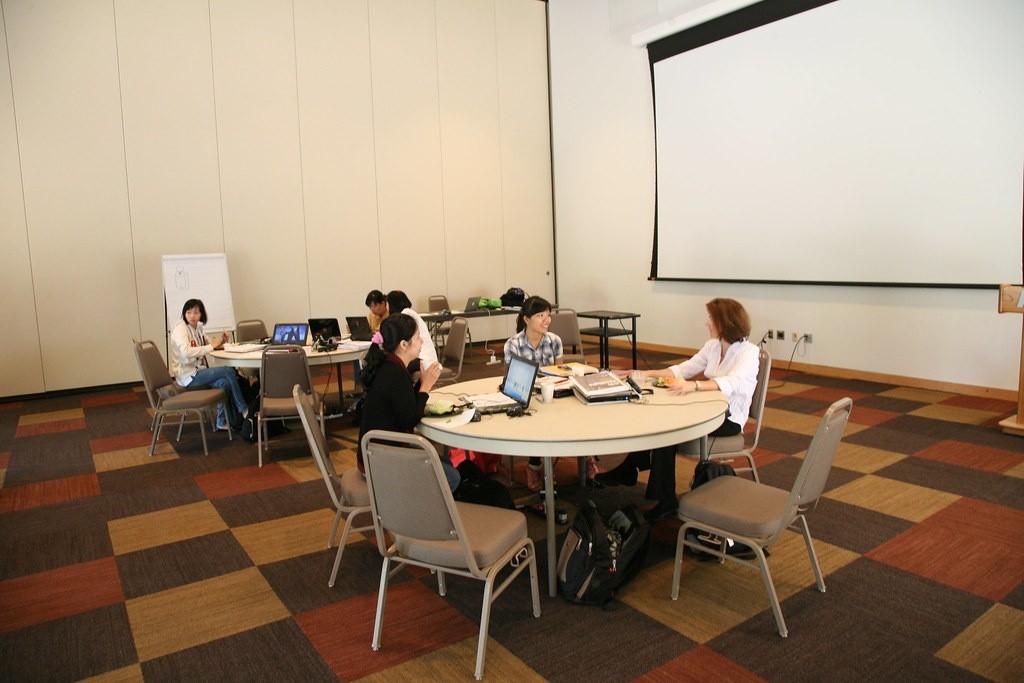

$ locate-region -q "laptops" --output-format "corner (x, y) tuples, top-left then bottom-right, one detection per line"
(457, 354), (539, 415)
(465, 296), (482, 312)
(308, 318), (342, 343)
(270, 323), (309, 346)
(346, 316), (374, 342)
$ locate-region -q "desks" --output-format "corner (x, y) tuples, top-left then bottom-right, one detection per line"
(417, 306), (549, 357)
(431, 367), (741, 594)
(575, 308), (638, 369)
(207, 339), (374, 440)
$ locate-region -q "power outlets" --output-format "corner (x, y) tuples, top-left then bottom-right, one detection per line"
(769, 330), (774, 338)
(777, 330), (784, 340)
(804, 334), (812, 343)
(792, 333), (797, 342)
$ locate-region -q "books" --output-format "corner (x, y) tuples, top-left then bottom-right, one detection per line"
(569, 370), (641, 406)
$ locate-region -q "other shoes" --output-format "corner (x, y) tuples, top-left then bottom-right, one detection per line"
(343, 391), (366, 399)
(526, 461), (544, 491)
(647, 496), (679, 520)
(347, 398), (362, 412)
(594, 460), (639, 487)
(586, 456), (599, 479)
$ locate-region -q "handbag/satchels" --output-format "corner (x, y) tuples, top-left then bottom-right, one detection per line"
(454, 458), (517, 510)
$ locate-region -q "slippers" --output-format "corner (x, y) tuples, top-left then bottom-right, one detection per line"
(216, 424), (233, 430)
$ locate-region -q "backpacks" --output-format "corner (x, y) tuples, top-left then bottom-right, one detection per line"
(683, 459), (772, 562)
(555, 499), (656, 607)
(241, 394), (292, 445)
(501, 288), (530, 307)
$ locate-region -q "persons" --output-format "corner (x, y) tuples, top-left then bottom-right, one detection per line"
(594, 298), (759, 518)
(360, 312), (461, 493)
(343, 289), (387, 398)
(171, 298), (250, 430)
(384, 290), (442, 371)
(503, 295), (599, 493)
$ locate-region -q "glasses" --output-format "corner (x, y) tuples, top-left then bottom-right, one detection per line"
(318, 345), (337, 352)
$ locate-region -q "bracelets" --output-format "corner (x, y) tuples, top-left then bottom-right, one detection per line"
(695, 381), (701, 391)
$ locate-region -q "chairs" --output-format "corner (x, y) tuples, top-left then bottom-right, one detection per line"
(136, 295), (856, 679)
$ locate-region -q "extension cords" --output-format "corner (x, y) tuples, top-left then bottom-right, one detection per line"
(524, 504), (569, 525)
(486, 359), (502, 365)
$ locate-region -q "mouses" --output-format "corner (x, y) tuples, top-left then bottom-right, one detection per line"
(470, 411), (481, 422)
